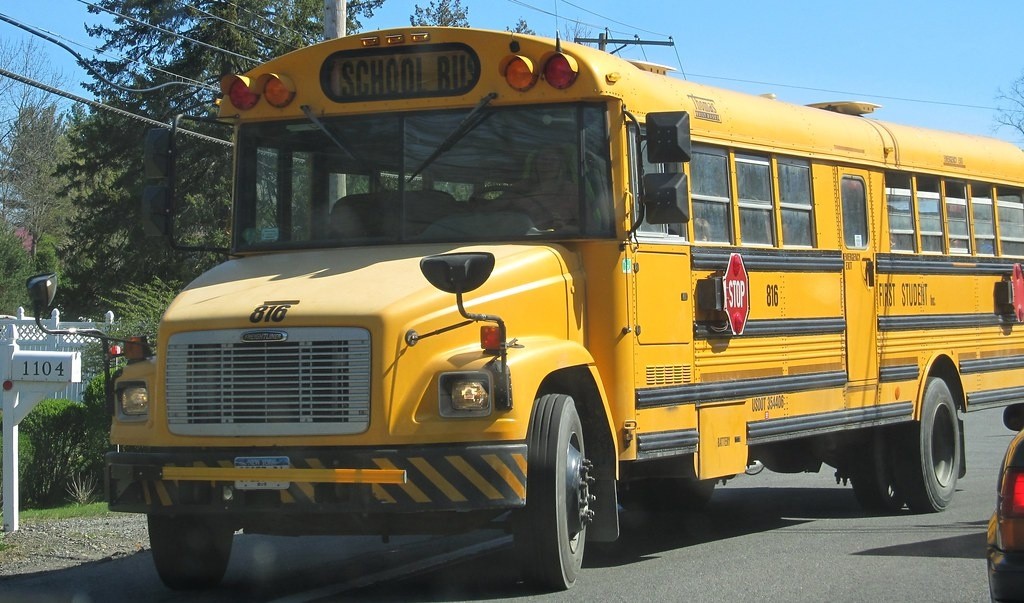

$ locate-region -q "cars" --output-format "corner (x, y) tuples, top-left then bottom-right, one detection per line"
(987, 403), (1024, 603)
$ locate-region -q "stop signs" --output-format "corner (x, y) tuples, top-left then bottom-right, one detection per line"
(723, 253), (750, 334)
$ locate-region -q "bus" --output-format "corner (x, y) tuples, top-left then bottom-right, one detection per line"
(27, 27), (1023, 589)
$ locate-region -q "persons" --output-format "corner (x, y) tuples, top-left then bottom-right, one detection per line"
(468, 144), (599, 233)
(694, 217), (711, 241)
